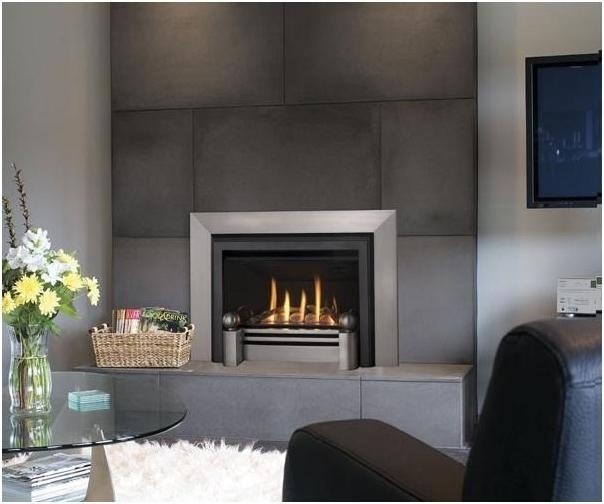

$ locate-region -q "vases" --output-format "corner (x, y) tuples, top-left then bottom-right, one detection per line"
(10, 412), (52, 447)
(7, 323), (53, 412)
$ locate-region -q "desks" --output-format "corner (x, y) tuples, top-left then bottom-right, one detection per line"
(2, 370), (188, 502)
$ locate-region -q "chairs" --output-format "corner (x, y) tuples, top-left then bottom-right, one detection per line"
(282, 316), (602, 501)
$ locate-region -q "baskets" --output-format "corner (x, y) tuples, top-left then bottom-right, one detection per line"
(88, 320), (195, 368)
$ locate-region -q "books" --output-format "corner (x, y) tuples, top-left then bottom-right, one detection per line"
(111, 305), (188, 334)
(2, 452), (91, 502)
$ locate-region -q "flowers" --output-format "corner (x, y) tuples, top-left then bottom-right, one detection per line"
(2, 160), (101, 410)
